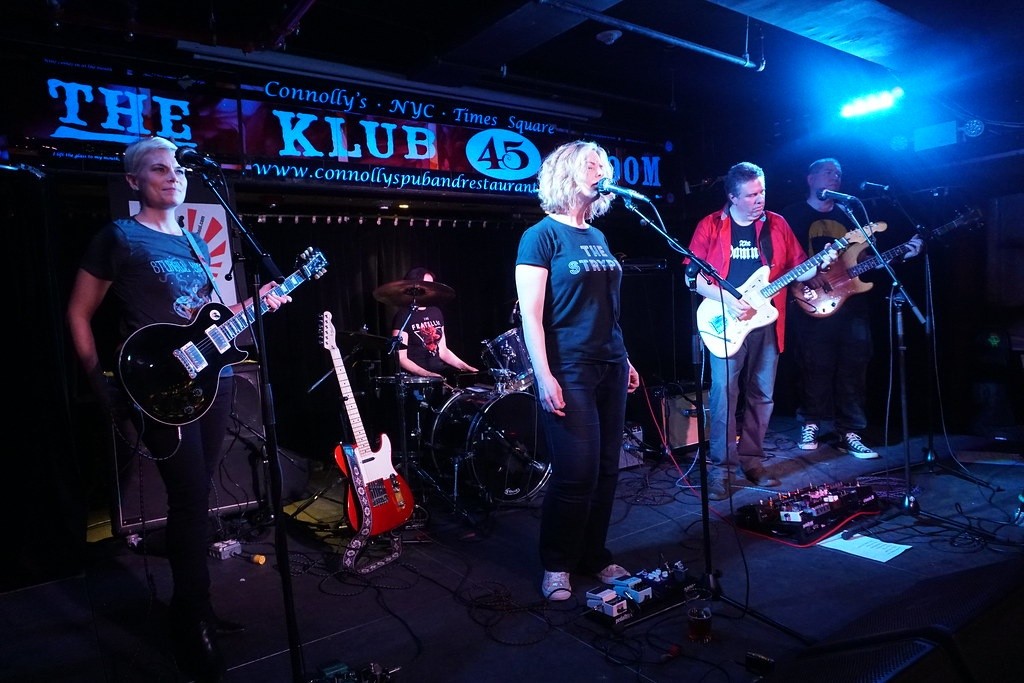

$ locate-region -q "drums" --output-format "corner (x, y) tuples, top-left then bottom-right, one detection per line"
(365, 374), (446, 456)
(428, 386), (554, 505)
(478, 327), (537, 391)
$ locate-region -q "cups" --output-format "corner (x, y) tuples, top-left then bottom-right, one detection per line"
(686, 588), (713, 643)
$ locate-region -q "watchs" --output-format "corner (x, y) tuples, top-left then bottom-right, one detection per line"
(817, 262), (831, 274)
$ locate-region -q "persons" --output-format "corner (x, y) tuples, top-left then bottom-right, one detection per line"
(779, 158), (924, 459)
(682, 161), (838, 501)
(391, 266), (479, 382)
(515, 140), (639, 601)
(69, 135), (293, 683)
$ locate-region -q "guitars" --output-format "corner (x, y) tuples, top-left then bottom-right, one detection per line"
(315, 309), (416, 539)
(116, 245), (330, 429)
(697, 219), (890, 358)
(792, 204), (988, 318)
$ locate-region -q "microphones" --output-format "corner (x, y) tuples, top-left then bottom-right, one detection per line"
(532, 461), (546, 472)
(597, 178), (652, 204)
(860, 182), (891, 192)
(682, 409), (698, 416)
(174, 146), (220, 170)
(821, 189), (856, 201)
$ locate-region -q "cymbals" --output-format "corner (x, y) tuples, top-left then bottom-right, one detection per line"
(336, 329), (409, 354)
(370, 279), (457, 308)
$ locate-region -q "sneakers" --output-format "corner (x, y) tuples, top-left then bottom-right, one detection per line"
(838, 432), (878, 459)
(542, 568), (572, 601)
(736, 464), (781, 486)
(798, 420), (821, 449)
(706, 478), (732, 500)
(592, 564), (633, 584)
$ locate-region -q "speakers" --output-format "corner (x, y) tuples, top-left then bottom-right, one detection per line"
(100, 356), (271, 535)
(757, 620), (976, 683)
(647, 381), (711, 456)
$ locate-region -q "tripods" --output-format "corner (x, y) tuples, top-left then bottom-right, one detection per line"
(614, 186), (1008, 645)
(393, 380), (479, 530)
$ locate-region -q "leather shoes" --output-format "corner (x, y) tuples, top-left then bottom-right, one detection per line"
(174, 600), (249, 638)
(182, 611), (229, 675)
